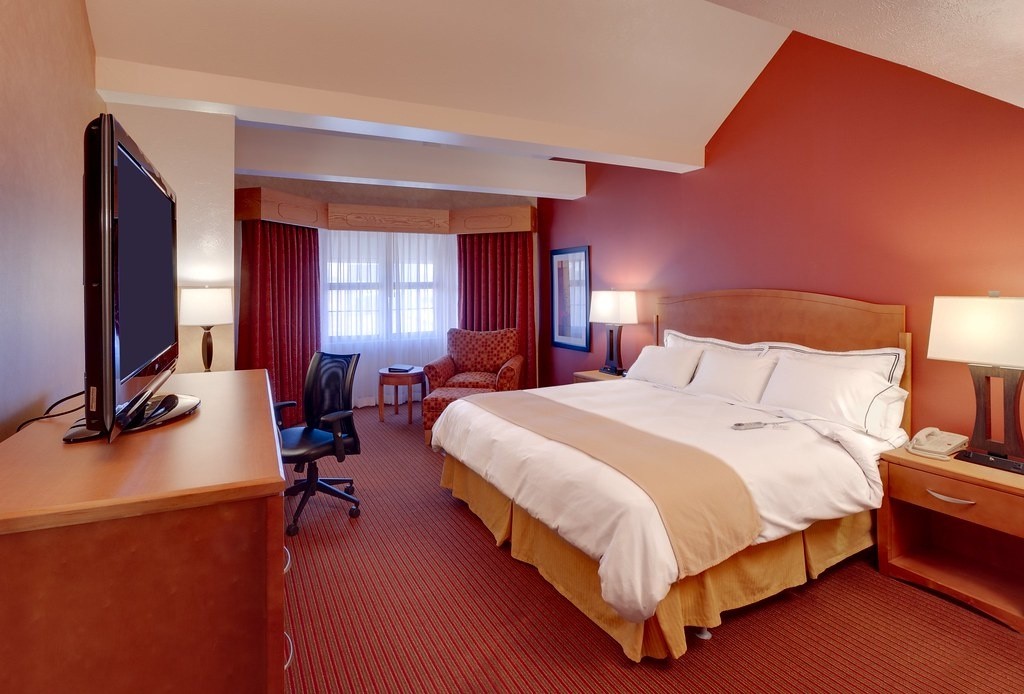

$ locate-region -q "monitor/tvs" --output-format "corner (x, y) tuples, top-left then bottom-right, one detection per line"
(64, 111), (203, 441)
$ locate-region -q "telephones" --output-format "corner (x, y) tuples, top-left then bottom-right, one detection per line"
(904, 426), (970, 457)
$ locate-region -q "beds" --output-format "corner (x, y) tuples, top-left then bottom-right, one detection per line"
(432, 288), (911, 663)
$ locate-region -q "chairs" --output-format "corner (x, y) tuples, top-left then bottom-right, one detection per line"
(274, 351), (360, 536)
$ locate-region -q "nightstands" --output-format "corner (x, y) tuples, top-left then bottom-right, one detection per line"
(877, 446), (1024, 635)
(574, 368), (626, 382)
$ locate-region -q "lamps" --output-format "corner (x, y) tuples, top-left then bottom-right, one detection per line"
(927, 291), (1024, 474)
(589, 291), (638, 375)
(178, 287), (233, 372)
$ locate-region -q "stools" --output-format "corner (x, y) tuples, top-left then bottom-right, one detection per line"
(422, 387), (496, 446)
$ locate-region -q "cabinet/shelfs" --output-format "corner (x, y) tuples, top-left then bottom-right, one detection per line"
(0, 368), (293, 694)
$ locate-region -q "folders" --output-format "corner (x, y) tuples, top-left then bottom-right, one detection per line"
(388, 363), (414, 372)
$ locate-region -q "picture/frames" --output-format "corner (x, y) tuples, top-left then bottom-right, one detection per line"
(550, 245), (590, 352)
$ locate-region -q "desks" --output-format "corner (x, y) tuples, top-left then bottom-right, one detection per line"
(378, 366), (426, 424)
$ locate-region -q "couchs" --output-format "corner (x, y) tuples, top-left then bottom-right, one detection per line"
(422, 328), (523, 393)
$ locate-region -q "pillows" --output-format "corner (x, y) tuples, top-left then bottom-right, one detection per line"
(664, 329), (769, 358)
(760, 355), (909, 441)
(625, 345), (704, 390)
(682, 350), (778, 405)
(763, 341), (906, 387)
